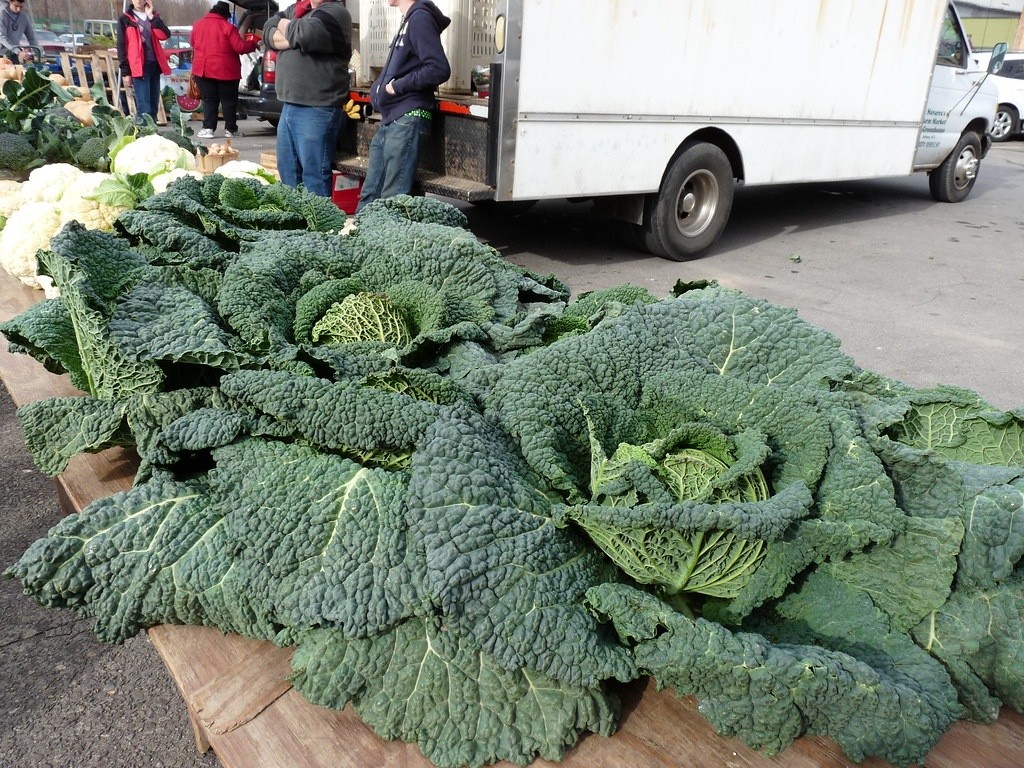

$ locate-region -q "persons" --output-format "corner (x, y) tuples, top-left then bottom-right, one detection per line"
(0, 0), (45, 65)
(263, 0), (353, 197)
(355, 0), (451, 217)
(189, 1), (256, 139)
(117, 0), (171, 126)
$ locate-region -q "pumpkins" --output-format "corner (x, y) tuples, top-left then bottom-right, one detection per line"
(0, 54), (125, 127)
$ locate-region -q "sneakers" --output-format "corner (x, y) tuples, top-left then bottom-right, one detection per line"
(225, 129), (245, 137)
(197, 127), (215, 139)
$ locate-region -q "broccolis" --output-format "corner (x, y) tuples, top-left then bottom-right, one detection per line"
(0, 105), (275, 300)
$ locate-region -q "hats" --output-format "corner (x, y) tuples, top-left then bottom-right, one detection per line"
(211, 1), (232, 18)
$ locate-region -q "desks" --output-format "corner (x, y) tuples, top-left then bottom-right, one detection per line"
(0, 262), (1024, 768)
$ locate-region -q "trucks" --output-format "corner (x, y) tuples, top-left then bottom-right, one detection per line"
(334, 0), (1006, 265)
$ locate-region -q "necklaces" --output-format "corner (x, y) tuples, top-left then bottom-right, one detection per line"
(3, 9), (21, 31)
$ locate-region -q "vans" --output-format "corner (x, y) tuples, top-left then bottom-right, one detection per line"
(220, 0), (300, 130)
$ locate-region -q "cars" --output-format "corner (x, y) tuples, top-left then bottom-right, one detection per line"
(968, 51), (1023, 143)
(21, 19), (196, 72)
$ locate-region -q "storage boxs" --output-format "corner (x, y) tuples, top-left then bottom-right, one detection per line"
(331, 172), (362, 215)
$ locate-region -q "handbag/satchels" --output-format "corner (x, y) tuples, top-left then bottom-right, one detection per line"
(188, 73), (202, 100)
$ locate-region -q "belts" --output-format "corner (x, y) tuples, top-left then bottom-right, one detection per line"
(404, 108), (432, 120)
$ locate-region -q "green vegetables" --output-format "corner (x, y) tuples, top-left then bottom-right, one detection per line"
(0, 175), (1024, 768)
